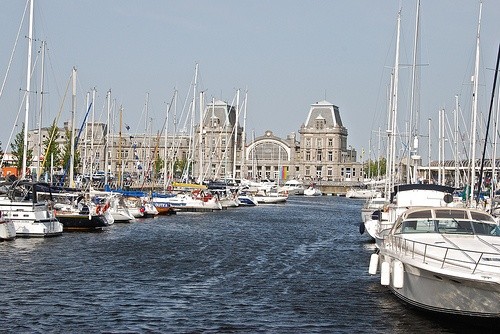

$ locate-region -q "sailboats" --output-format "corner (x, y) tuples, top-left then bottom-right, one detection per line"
(345, 0), (500, 244)
(0, 0), (324, 239)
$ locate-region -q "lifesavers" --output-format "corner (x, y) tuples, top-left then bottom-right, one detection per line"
(103, 204), (107, 212)
(106, 200), (110, 208)
(96, 205), (101, 214)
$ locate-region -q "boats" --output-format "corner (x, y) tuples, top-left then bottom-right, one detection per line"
(369, 205), (500, 320)
